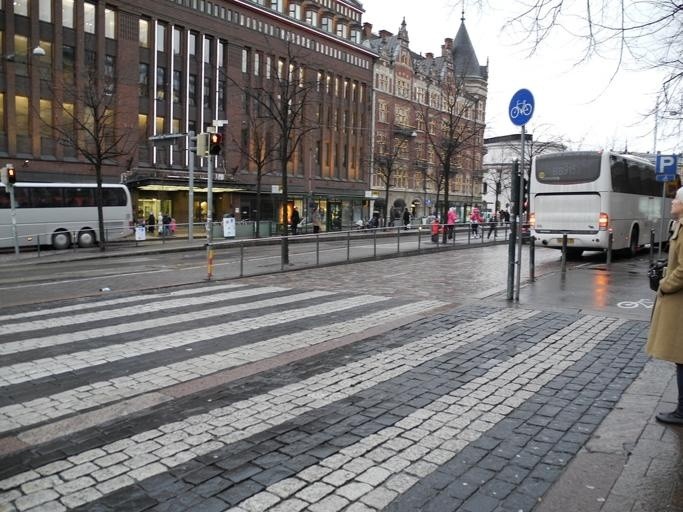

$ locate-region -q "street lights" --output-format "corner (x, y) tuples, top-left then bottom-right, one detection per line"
(384, 129), (418, 228)
(2, 43), (47, 64)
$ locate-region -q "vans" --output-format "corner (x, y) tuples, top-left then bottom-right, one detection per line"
(517, 213), (529, 244)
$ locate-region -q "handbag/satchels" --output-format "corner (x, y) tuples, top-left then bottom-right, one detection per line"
(648, 257), (668, 292)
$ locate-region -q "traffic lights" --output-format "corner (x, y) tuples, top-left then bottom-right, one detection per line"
(208, 130), (222, 156)
(188, 131), (207, 158)
(513, 174), (528, 216)
(5, 164), (17, 185)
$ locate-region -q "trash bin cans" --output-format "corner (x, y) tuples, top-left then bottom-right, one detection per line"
(222, 213), (236, 239)
(134, 217), (146, 241)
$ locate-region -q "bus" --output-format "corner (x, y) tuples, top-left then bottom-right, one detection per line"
(0, 180), (135, 252)
(526, 152), (681, 261)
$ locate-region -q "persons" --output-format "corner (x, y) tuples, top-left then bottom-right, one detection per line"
(402, 207), (411, 230)
(157, 211), (163, 236)
(356, 213), (385, 232)
(169, 214), (176, 233)
(428, 203), (509, 242)
(291, 205), (300, 235)
(312, 208), (321, 234)
(163, 212), (171, 231)
(645, 186), (683, 423)
(146, 212), (155, 234)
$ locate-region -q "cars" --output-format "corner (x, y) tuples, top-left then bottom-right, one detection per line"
(279, 214), (313, 235)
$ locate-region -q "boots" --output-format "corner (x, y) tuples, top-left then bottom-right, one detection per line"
(655, 365), (683, 425)
